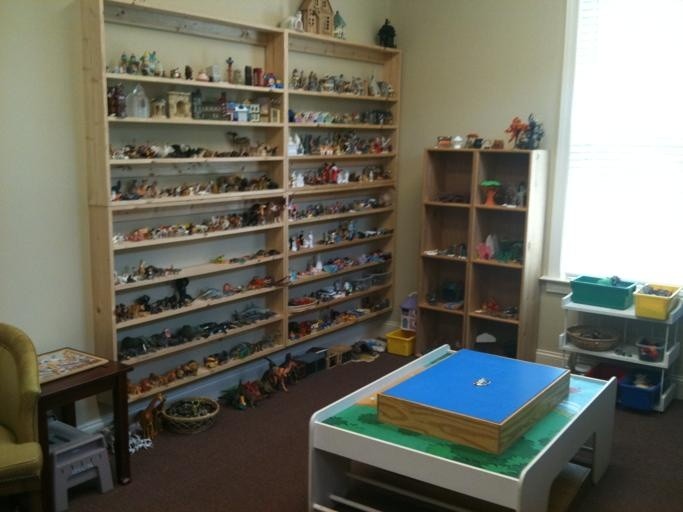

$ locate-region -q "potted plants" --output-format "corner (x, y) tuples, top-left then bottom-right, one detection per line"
(164, 397), (218, 434)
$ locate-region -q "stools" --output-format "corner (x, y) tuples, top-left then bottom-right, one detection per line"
(35, 346), (132, 512)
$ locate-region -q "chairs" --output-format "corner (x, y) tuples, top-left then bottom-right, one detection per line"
(1, 324), (42, 512)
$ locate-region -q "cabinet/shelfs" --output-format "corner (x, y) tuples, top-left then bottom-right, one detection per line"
(559, 294), (683, 414)
(84, 1), (401, 406)
(415, 149), (549, 363)
